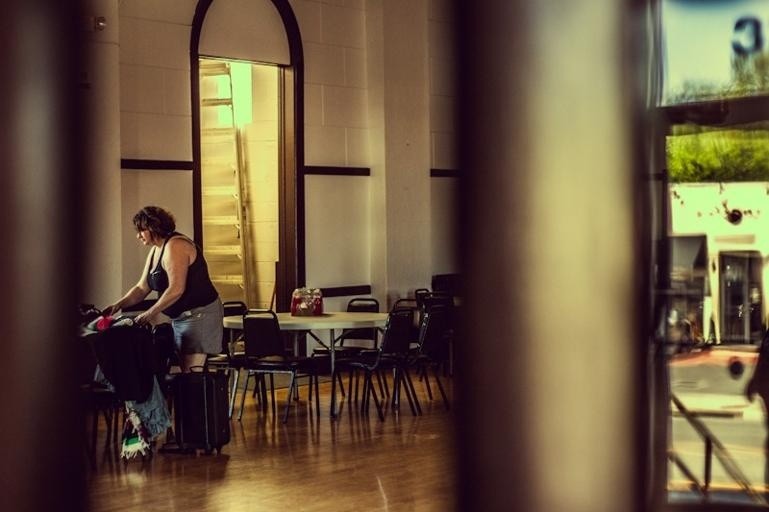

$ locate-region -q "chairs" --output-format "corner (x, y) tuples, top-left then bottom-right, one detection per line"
(220, 301), (296, 399)
(83, 307), (173, 471)
(309, 290), (455, 421)
(238, 309), (320, 423)
(207, 326), (268, 418)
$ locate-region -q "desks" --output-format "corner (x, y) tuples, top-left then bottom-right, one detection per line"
(222, 314), (390, 420)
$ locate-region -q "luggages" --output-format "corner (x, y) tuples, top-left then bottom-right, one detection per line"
(168, 364), (231, 454)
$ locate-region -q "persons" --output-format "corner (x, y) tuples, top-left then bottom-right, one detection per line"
(99, 205), (225, 456)
